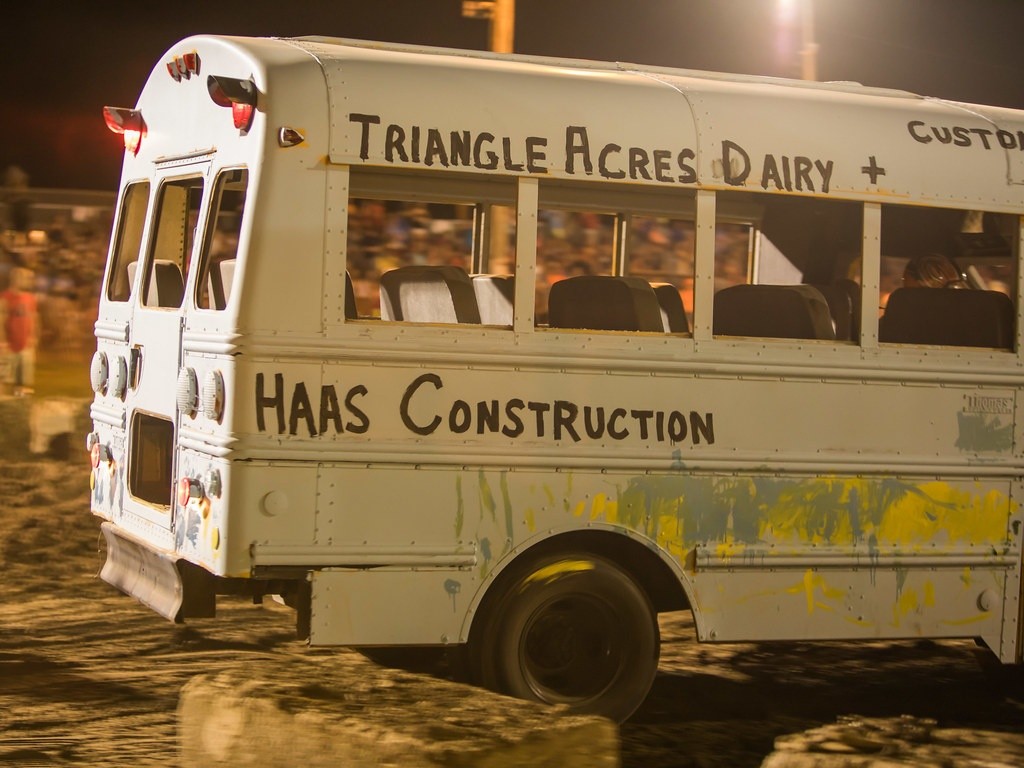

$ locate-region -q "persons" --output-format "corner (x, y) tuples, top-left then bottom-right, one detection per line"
(0, 216), (111, 330)
(905, 253), (967, 289)
(344, 201), (748, 322)
(0, 267), (38, 395)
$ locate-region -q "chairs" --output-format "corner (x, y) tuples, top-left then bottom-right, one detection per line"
(127, 251), (1016, 345)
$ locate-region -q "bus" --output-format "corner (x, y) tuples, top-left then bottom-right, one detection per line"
(87, 34), (1024, 727)
